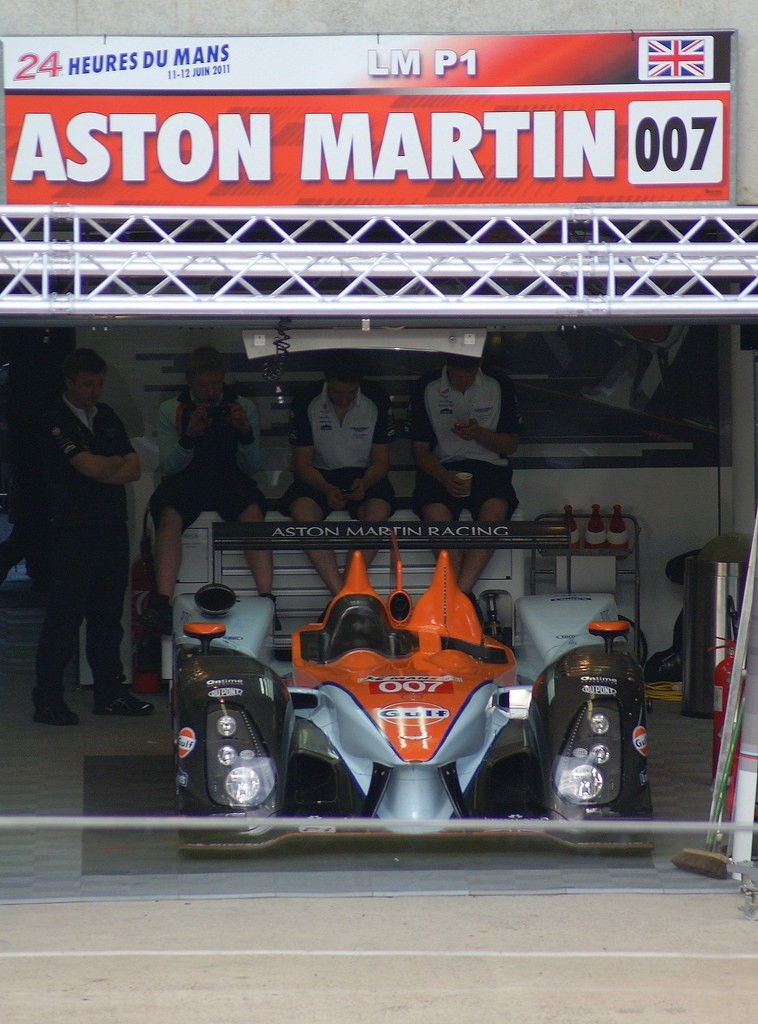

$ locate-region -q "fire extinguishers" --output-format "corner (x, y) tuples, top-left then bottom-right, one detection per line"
(703, 635), (747, 788)
(131, 508), (159, 694)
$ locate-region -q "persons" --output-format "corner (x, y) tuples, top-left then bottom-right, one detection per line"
(280, 348), (397, 603)
(142, 346), (286, 635)
(30, 347), (153, 725)
(2, 340), (67, 603)
(403, 346), (524, 620)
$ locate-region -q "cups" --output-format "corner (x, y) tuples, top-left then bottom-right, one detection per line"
(455, 472), (472, 497)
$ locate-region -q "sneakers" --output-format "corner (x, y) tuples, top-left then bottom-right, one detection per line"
(31, 685), (80, 725)
(90, 676), (154, 716)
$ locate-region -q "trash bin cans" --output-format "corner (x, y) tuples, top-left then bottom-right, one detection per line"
(682, 556), (748, 720)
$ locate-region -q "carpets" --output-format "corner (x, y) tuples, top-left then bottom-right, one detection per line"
(82, 752), (656, 876)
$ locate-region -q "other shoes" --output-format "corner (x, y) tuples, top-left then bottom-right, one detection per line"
(465, 590), (482, 625)
(139, 593), (174, 630)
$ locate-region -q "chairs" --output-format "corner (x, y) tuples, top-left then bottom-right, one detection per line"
(326, 609), (387, 660)
